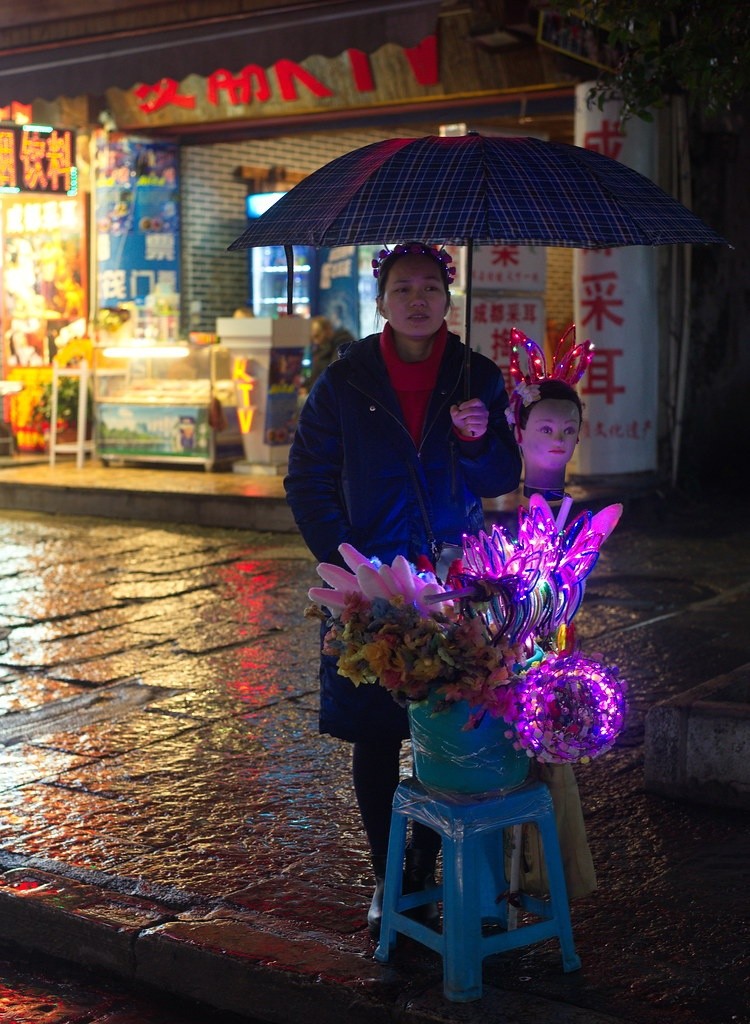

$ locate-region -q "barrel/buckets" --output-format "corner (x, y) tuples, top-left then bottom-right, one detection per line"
(406, 683), (549, 796)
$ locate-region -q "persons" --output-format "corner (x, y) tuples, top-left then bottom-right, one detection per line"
(300, 315), (354, 396)
(283, 238), (522, 931)
(514, 380), (583, 490)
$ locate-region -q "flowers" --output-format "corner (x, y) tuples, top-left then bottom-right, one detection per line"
(301, 587), (629, 767)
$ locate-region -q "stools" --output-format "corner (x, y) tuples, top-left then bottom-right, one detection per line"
(375, 777), (581, 1001)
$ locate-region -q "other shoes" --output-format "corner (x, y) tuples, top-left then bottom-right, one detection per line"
(406, 878), (438, 930)
(367, 882), (403, 947)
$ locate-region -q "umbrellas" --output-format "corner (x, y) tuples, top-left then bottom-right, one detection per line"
(227, 127), (736, 403)
